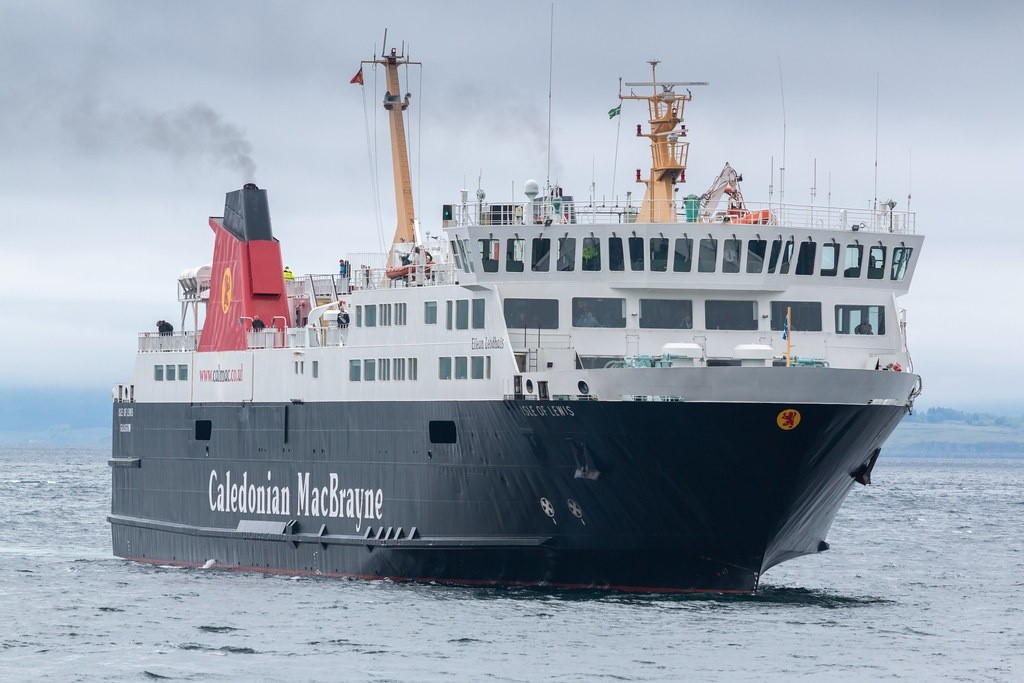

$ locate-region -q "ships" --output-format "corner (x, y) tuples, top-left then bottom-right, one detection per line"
(106, 29), (925, 600)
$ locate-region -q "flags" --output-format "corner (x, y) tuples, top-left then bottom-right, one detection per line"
(351, 67), (364, 86)
(608, 105), (621, 119)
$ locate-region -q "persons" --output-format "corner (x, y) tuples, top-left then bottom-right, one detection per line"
(157, 320), (174, 352)
(338, 259), (352, 295)
(284, 266), (298, 298)
(336, 306), (350, 346)
(868, 250), (876, 268)
(252, 316), (266, 349)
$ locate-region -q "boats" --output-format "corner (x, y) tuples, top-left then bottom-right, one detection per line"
(729, 209), (776, 225)
(385, 258), (437, 280)
